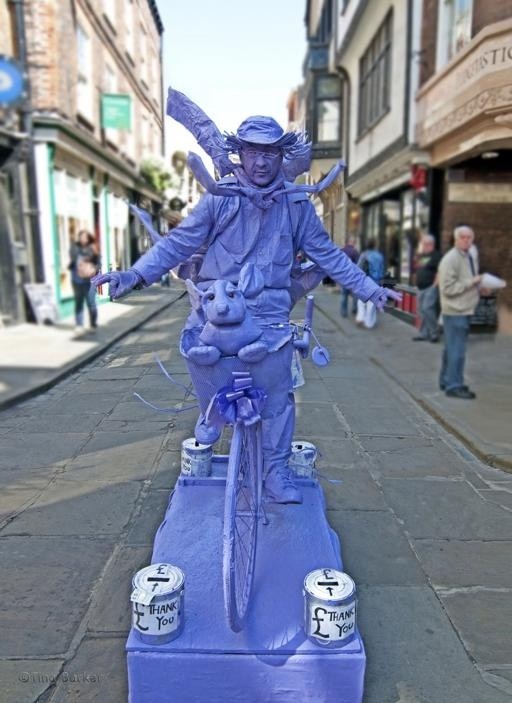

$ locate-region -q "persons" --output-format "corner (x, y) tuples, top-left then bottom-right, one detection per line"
(412, 226), (493, 399)
(66, 230), (104, 330)
(92, 116), (405, 506)
(339, 237), (386, 330)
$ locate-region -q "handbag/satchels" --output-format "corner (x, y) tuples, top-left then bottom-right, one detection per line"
(76, 256), (96, 279)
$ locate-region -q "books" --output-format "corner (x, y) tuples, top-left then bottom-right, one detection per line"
(477, 272), (507, 293)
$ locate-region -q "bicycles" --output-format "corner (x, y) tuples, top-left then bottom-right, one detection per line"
(179, 295), (329, 632)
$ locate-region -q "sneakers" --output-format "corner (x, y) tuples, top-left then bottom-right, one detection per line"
(72, 324), (98, 337)
(412, 336), (440, 343)
(438, 381), (475, 399)
